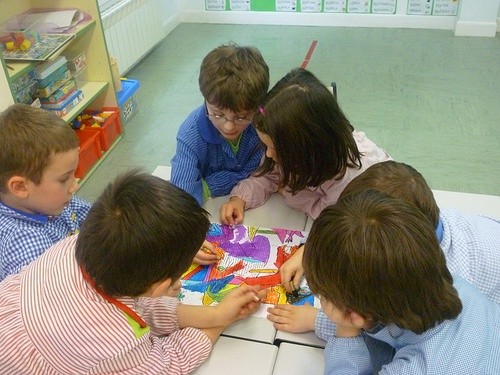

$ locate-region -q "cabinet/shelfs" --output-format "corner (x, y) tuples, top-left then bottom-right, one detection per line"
(0, 0), (125, 198)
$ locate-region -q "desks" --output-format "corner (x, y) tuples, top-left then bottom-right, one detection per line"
(150, 164), (500, 375)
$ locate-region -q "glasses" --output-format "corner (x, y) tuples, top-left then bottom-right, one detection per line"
(204, 101), (255, 125)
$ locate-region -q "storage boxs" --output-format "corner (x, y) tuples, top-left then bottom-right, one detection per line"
(11, 50), (142, 180)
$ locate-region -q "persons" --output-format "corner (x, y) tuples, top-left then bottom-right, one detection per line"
(0, 168), (260, 375)
(170, 44), (500, 375)
(0, 103), (92, 281)
